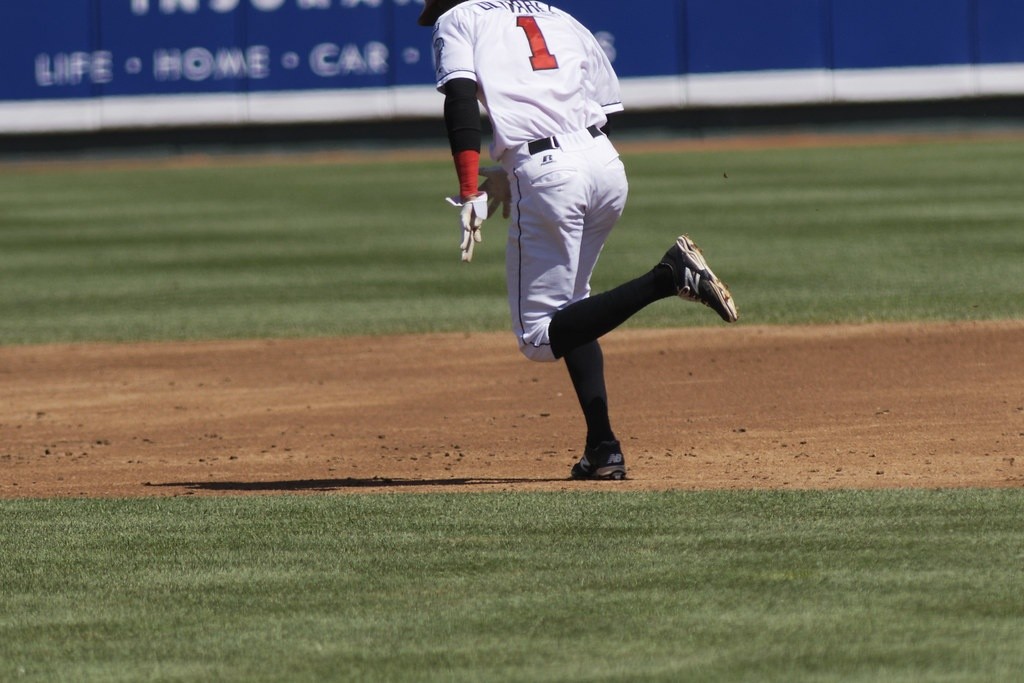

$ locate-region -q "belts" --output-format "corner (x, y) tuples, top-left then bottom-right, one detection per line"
(528, 125), (602, 155)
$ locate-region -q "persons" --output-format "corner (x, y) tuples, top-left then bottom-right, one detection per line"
(418, 0), (740, 481)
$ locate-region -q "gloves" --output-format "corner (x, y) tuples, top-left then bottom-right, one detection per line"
(445, 191), (489, 262)
(477, 167), (511, 219)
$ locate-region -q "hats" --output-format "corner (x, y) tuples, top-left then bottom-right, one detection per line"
(418, 0), (465, 26)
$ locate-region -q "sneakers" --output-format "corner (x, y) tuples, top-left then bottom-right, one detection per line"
(660, 234), (738, 323)
(572, 441), (625, 480)
